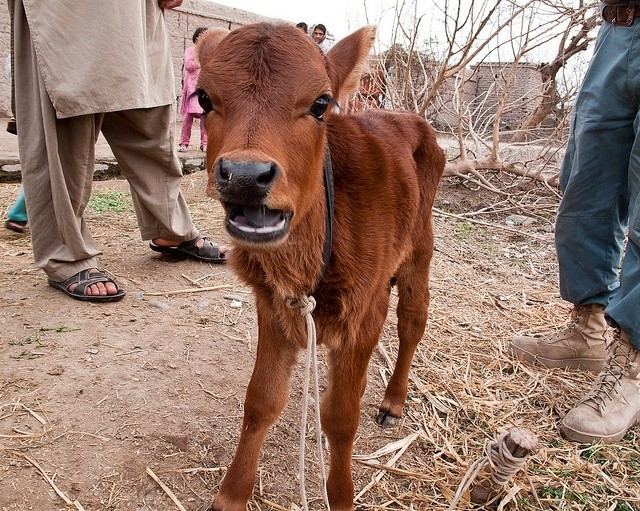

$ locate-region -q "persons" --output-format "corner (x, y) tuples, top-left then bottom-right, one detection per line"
(297, 22), (309, 33)
(4, 184), (28, 233)
(7, 1), (231, 302)
(310, 23), (331, 52)
(178, 27), (212, 152)
(510, 1), (640, 445)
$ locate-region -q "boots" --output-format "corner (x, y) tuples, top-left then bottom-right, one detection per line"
(509, 304), (611, 376)
(562, 328), (639, 444)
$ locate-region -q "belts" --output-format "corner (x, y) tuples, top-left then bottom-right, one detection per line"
(602, 2), (640, 26)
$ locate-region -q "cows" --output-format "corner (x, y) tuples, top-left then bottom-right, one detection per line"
(194, 18), (448, 511)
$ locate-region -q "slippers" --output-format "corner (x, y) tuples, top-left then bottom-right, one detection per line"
(47, 266), (126, 302)
(149, 231), (229, 263)
